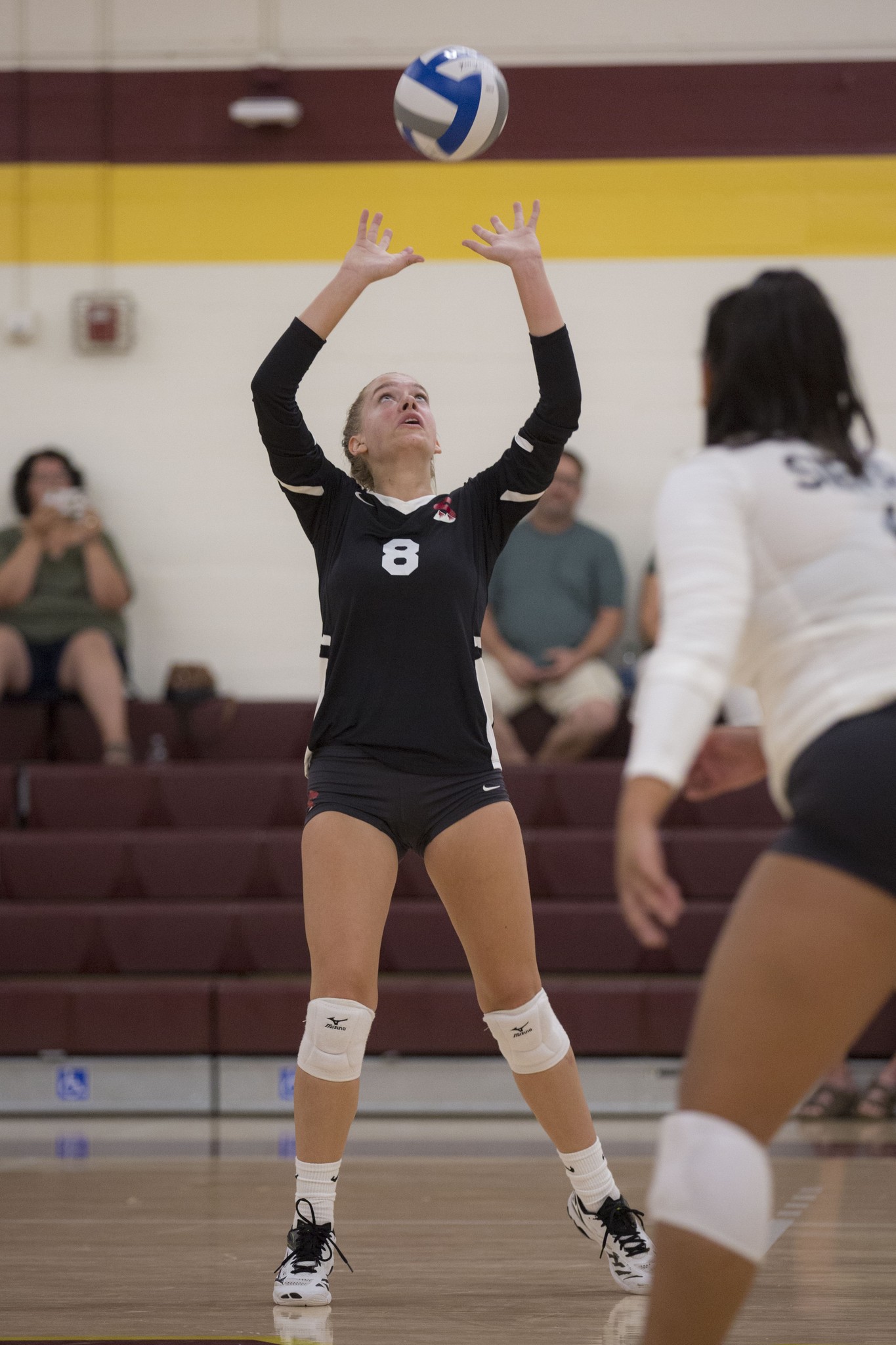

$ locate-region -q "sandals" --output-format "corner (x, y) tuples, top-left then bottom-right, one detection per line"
(794, 1075), (896, 1120)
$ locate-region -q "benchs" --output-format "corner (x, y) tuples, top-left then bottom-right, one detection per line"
(2, 666), (896, 1117)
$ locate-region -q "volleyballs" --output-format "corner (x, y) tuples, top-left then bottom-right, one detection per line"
(394, 43), (510, 163)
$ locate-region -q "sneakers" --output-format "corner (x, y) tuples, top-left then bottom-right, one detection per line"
(604, 1295), (649, 1344)
(273, 1303), (334, 1345)
(272, 1221), (336, 1306)
(566, 1191), (657, 1294)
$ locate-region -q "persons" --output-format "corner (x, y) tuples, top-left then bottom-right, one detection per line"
(610, 267), (896, 1344)
(0, 447), (137, 768)
(481, 449), (629, 772)
(629, 549), (764, 727)
(248, 201), (658, 1311)
